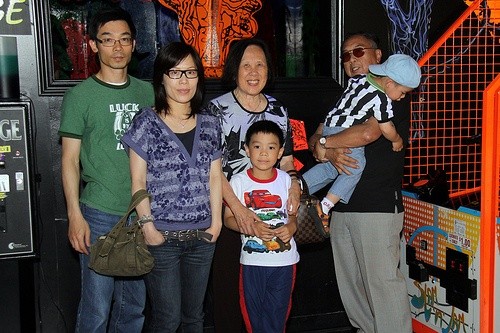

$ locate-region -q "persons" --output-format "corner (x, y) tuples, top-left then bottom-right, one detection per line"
(120, 42), (222, 333)
(57, 8), (155, 333)
(202, 37), (300, 333)
(296, 54), (421, 237)
(225, 120), (300, 333)
(309, 35), (413, 333)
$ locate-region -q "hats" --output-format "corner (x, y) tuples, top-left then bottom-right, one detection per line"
(368, 54), (422, 88)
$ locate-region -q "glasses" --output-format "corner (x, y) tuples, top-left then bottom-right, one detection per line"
(163, 69), (199, 79)
(94, 37), (132, 47)
(340, 48), (377, 62)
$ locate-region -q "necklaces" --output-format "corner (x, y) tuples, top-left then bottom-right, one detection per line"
(167, 113), (193, 128)
(250, 96), (261, 112)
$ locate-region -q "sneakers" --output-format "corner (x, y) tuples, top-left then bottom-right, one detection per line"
(296, 177), (309, 201)
(306, 200), (330, 238)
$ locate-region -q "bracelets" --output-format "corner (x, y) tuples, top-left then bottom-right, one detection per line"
(137, 215), (154, 227)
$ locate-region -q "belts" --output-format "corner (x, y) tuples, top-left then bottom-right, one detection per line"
(158, 228), (214, 241)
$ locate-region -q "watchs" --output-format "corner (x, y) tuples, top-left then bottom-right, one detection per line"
(319, 135), (329, 149)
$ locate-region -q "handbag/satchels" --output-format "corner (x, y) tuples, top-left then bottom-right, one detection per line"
(88, 189), (155, 277)
